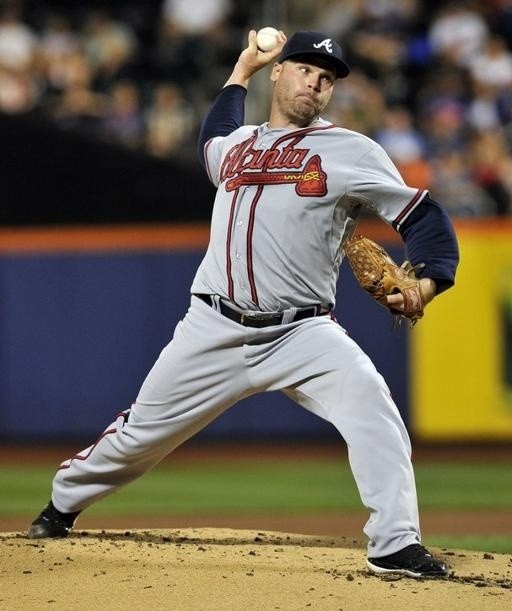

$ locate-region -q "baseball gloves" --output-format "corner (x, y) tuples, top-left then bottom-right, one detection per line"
(343, 234), (421, 328)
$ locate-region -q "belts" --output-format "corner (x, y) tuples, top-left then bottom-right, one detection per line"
(192, 291), (334, 329)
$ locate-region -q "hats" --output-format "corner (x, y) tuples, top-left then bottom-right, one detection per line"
(278, 30), (351, 79)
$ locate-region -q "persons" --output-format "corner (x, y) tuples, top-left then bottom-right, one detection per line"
(28, 29), (460, 578)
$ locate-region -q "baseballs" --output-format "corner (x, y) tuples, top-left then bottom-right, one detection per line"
(256, 26), (279, 51)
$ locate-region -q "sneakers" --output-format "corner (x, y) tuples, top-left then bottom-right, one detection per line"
(25, 498), (84, 541)
(365, 543), (456, 580)
